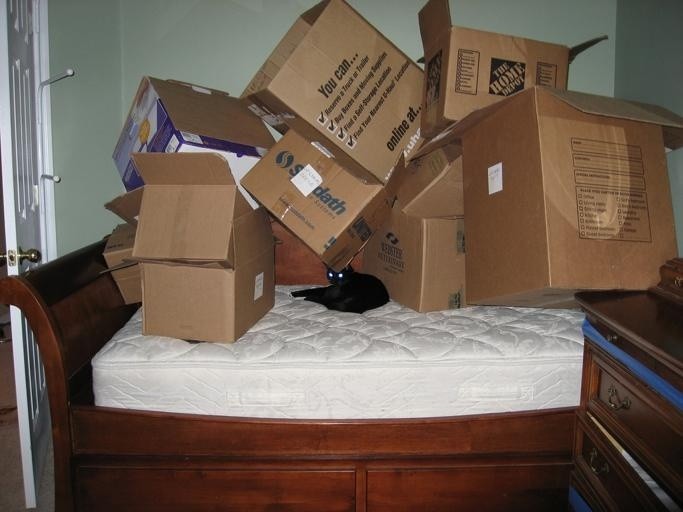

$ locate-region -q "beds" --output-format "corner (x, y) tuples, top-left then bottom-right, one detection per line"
(3, 220), (583, 512)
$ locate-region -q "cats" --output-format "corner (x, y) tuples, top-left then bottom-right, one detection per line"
(290, 261), (391, 315)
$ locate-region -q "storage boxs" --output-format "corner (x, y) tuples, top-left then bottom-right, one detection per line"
(99, 3), (682, 345)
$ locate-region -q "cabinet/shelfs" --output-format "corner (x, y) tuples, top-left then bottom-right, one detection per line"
(572, 287), (683, 510)
